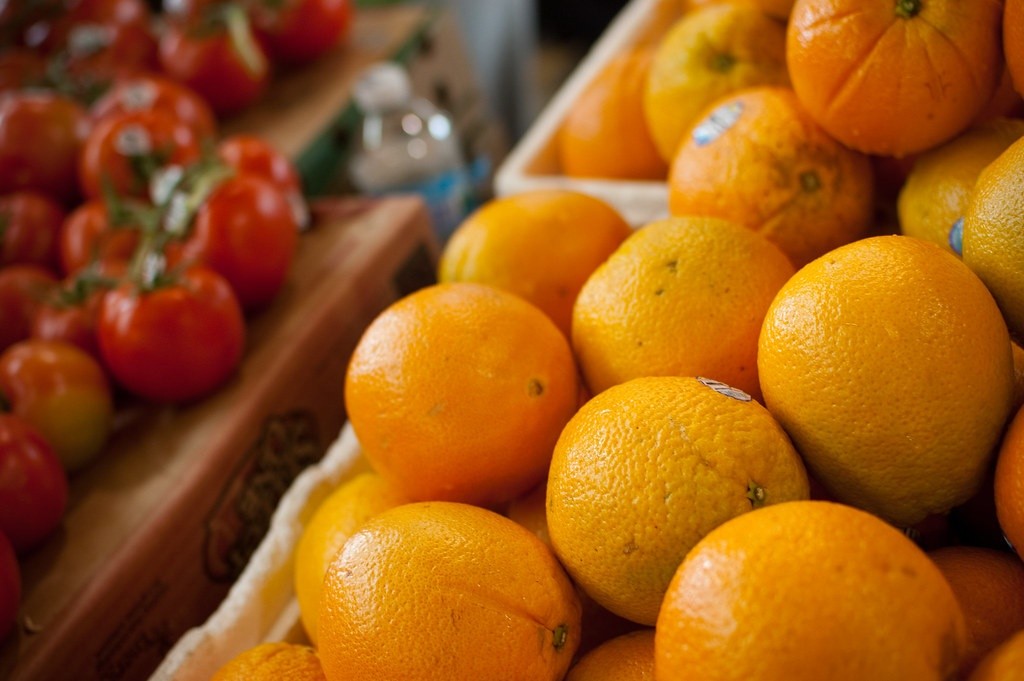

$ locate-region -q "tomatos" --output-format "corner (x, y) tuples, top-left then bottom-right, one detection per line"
(0, 0), (350, 645)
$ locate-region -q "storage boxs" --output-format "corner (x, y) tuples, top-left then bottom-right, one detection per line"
(494, 2), (673, 214)
(3, 195), (450, 679)
(229, 4), (507, 232)
(147, 412), (372, 675)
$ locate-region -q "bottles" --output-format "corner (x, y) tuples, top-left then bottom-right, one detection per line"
(345, 59), (478, 242)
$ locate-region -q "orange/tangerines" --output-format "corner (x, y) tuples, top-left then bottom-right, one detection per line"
(209, 2), (1023, 681)
